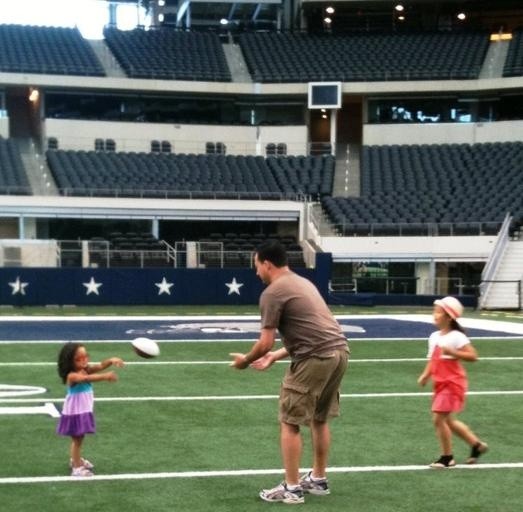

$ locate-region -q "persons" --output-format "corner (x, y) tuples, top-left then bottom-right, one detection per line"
(56, 341), (124, 479)
(417, 295), (489, 467)
(229, 238), (352, 505)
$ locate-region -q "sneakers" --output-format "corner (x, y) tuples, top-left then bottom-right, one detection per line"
(69, 457), (94, 468)
(466, 441), (488, 464)
(71, 465), (93, 476)
(297, 471), (330, 496)
(259, 481), (305, 504)
(428, 455), (456, 468)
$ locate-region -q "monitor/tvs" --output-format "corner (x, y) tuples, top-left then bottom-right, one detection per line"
(308, 82), (343, 110)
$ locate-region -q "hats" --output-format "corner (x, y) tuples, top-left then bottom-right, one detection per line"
(433, 295), (465, 322)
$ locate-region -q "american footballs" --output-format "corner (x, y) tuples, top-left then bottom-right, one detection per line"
(129, 338), (159, 358)
